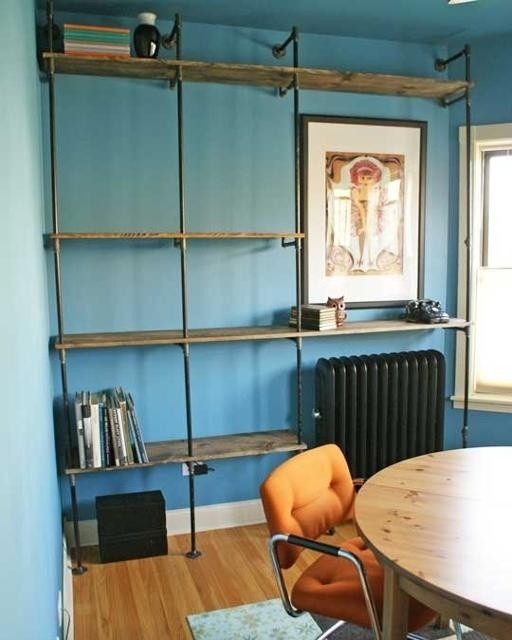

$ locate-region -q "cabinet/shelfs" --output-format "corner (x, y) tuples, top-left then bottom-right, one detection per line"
(41, 4), (483, 583)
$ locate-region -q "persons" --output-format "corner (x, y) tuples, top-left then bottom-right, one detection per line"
(345, 158), (390, 267)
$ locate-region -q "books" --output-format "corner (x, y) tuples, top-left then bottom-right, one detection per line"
(73, 385), (151, 468)
(288, 305), (337, 331)
(61, 23), (131, 57)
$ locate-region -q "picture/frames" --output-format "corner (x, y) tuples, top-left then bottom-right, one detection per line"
(300, 108), (433, 314)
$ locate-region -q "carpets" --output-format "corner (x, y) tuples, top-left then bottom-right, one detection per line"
(183, 587), (486, 640)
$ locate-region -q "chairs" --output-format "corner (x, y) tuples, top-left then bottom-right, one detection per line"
(258, 442), (462, 640)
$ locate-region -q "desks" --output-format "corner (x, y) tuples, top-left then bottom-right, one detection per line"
(352, 445), (512, 640)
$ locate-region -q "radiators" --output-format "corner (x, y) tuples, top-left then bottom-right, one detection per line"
(314, 350), (448, 481)
